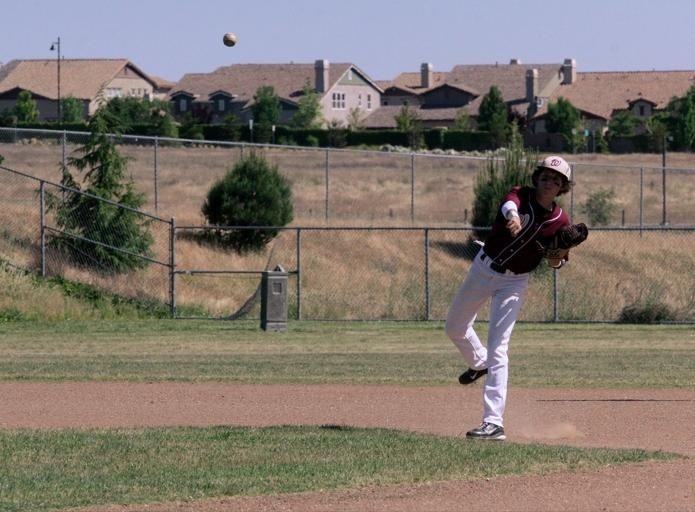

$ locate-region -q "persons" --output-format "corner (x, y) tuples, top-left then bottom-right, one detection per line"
(445, 155), (589, 440)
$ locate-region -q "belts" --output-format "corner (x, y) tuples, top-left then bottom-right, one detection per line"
(479, 253), (523, 276)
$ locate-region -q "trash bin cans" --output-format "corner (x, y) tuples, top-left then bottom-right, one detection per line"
(261, 271), (290, 331)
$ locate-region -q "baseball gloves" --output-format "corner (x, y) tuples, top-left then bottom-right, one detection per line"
(536, 223), (588, 258)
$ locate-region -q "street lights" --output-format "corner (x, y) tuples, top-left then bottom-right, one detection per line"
(49, 36), (61, 145)
(660, 134), (674, 225)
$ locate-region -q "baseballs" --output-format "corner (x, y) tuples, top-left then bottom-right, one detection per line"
(223, 32), (237, 46)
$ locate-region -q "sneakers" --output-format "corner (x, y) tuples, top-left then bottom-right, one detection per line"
(458, 366), (489, 385)
(466, 420), (506, 441)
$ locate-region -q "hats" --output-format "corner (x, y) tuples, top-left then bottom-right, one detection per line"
(537, 155), (572, 183)
